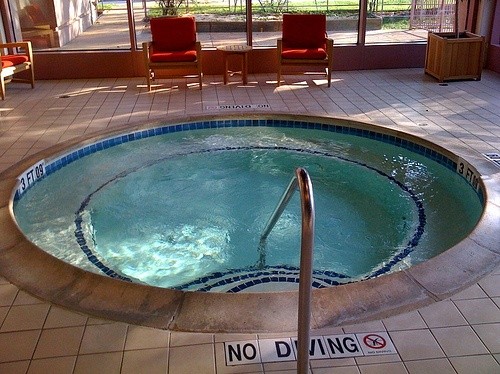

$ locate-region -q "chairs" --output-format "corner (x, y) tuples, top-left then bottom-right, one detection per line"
(0, 31), (34, 100)
(142, 15), (202, 91)
(277, 14), (334, 88)
(17, 4), (60, 48)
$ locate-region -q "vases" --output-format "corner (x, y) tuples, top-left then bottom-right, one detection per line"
(424, 30), (487, 83)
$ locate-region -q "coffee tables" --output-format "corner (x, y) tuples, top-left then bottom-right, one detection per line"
(217, 44), (252, 86)
